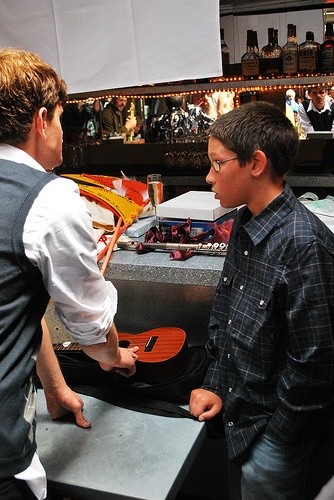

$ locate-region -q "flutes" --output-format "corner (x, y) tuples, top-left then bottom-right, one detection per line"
(116, 233), (229, 256)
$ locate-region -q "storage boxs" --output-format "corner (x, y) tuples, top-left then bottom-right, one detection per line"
(156, 190), (238, 244)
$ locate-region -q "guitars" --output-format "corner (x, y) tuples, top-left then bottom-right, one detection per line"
(50, 326), (190, 375)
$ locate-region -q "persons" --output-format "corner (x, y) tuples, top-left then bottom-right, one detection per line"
(58, 85), (334, 142)
(186, 101), (334, 500)
(0, 47), (140, 500)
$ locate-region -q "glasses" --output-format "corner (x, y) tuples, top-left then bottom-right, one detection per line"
(210, 156), (244, 172)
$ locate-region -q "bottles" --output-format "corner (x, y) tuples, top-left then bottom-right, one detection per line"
(220, 24), (334, 80)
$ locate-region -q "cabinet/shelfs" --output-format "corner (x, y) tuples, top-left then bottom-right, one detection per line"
(51, 0), (334, 98)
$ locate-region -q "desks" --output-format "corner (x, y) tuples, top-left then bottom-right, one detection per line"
(36, 279), (241, 500)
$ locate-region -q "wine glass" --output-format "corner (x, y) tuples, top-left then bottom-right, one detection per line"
(148, 174), (163, 228)
